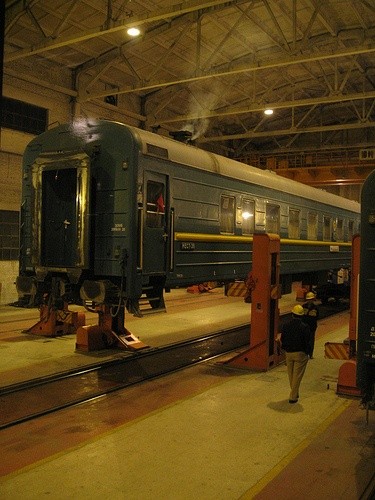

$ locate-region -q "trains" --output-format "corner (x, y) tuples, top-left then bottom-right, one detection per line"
(17, 118), (361, 310)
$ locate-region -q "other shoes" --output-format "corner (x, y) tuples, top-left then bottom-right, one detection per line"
(310, 356), (314, 359)
(289, 400), (298, 403)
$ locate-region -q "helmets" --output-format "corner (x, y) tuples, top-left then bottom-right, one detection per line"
(305, 292), (315, 299)
(292, 305), (304, 315)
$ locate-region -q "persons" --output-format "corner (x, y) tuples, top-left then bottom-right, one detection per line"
(301, 292), (320, 360)
(281, 304), (315, 404)
